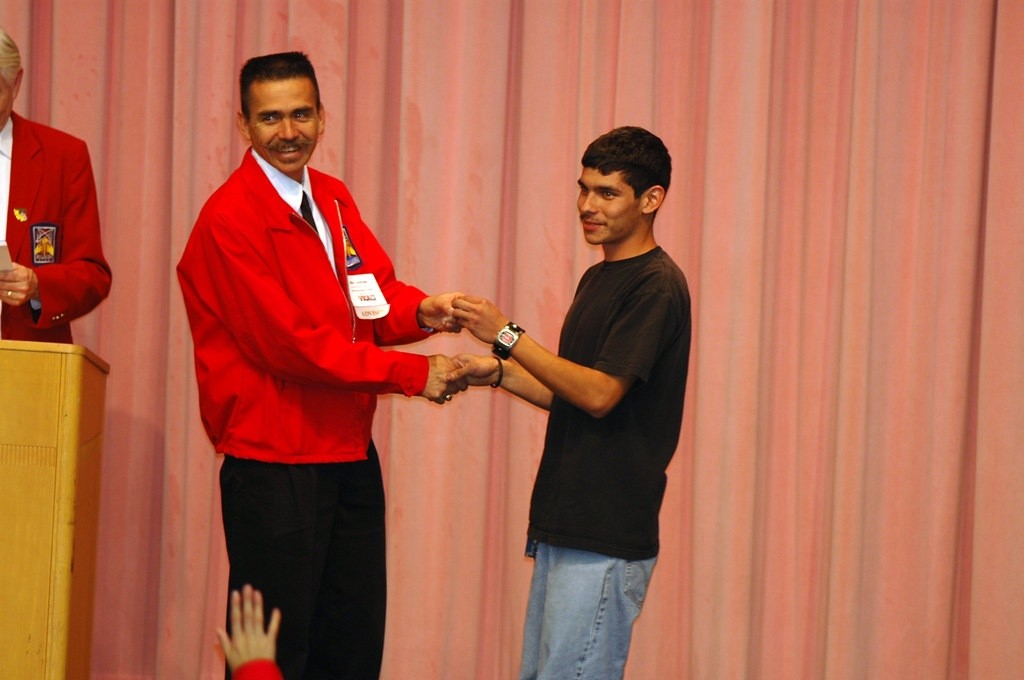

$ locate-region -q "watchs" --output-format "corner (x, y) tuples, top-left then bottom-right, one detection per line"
(492, 322), (525, 360)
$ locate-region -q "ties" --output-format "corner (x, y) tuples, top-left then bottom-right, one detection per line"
(300, 191), (318, 233)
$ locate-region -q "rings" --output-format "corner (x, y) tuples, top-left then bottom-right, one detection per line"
(444, 394), (451, 401)
(8, 291), (12, 298)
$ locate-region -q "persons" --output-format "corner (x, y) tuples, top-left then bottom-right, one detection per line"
(446, 126), (692, 680)
(176, 50), (468, 680)
(0, 28), (112, 344)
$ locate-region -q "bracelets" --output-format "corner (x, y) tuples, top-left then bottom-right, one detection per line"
(490, 357), (503, 388)
